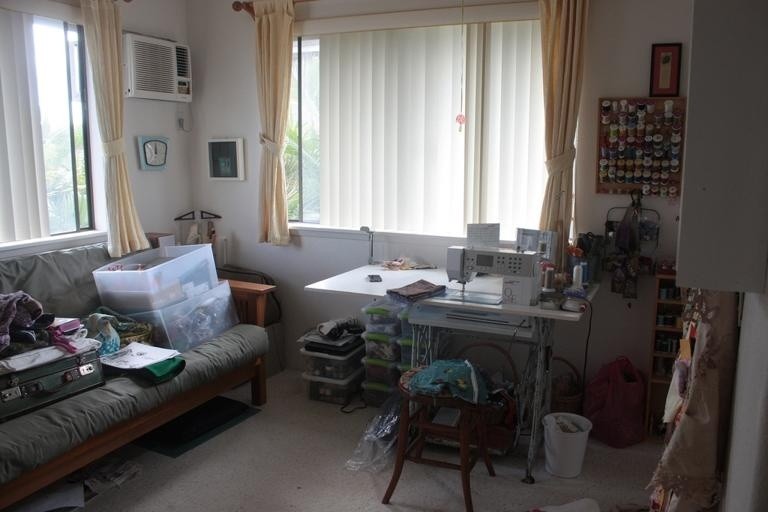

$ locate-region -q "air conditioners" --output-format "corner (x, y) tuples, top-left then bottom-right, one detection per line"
(122, 31), (193, 103)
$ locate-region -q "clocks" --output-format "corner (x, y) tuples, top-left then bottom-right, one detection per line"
(134, 134), (173, 173)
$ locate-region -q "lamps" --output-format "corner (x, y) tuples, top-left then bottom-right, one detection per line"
(358, 225), (376, 265)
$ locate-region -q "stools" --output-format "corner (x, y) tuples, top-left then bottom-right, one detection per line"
(380, 361), (508, 512)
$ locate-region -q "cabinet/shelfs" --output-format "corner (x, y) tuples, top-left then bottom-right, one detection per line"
(643, 272), (686, 441)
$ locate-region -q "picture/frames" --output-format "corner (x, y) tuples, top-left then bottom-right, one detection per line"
(206, 136), (245, 182)
(648, 42), (682, 97)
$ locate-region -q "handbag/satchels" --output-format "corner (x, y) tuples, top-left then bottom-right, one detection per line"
(48, 316), (81, 340)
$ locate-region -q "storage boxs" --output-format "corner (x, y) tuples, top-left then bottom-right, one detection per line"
(91, 242), (219, 315)
(298, 301), (426, 409)
(126, 278), (240, 355)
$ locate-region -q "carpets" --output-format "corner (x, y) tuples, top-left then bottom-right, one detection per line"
(130, 392), (262, 459)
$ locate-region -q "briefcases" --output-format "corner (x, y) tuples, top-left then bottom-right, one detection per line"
(1, 348), (106, 421)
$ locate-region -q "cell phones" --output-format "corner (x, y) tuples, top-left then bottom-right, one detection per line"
(369, 274), (381, 282)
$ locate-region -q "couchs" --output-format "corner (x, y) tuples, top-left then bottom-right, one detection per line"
(1, 231), (277, 510)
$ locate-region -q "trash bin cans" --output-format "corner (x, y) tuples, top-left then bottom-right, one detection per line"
(542, 412), (593, 478)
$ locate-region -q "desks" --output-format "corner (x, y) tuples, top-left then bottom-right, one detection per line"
(303, 264), (602, 485)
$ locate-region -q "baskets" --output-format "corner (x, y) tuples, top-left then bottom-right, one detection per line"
(119, 321), (153, 350)
(550, 358), (582, 414)
